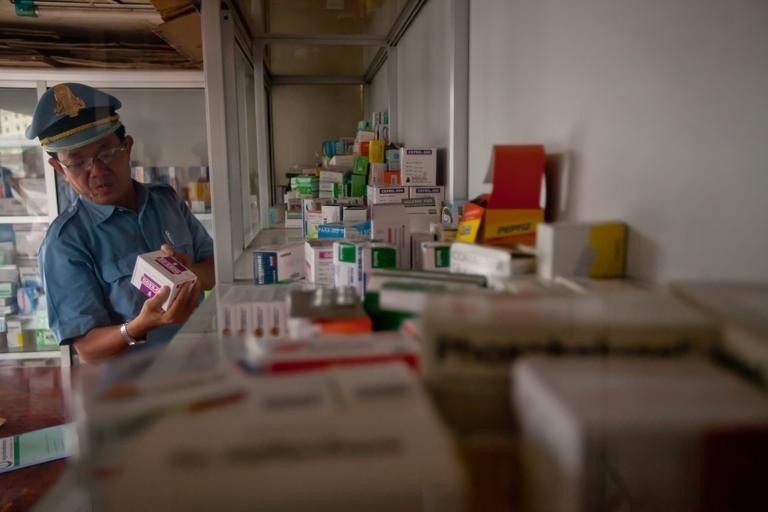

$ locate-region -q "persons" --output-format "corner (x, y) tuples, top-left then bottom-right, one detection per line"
(24, 82), (216, 380)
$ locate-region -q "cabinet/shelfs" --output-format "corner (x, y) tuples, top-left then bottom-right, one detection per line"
(0, 69), (271, 362)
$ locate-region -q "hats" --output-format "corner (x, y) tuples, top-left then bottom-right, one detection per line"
(25, 82), (121, 152)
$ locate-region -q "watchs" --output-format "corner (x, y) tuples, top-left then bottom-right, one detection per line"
(119, 319), (149, 346)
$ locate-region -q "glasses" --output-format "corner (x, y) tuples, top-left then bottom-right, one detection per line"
(57, 148), (127, 172)
